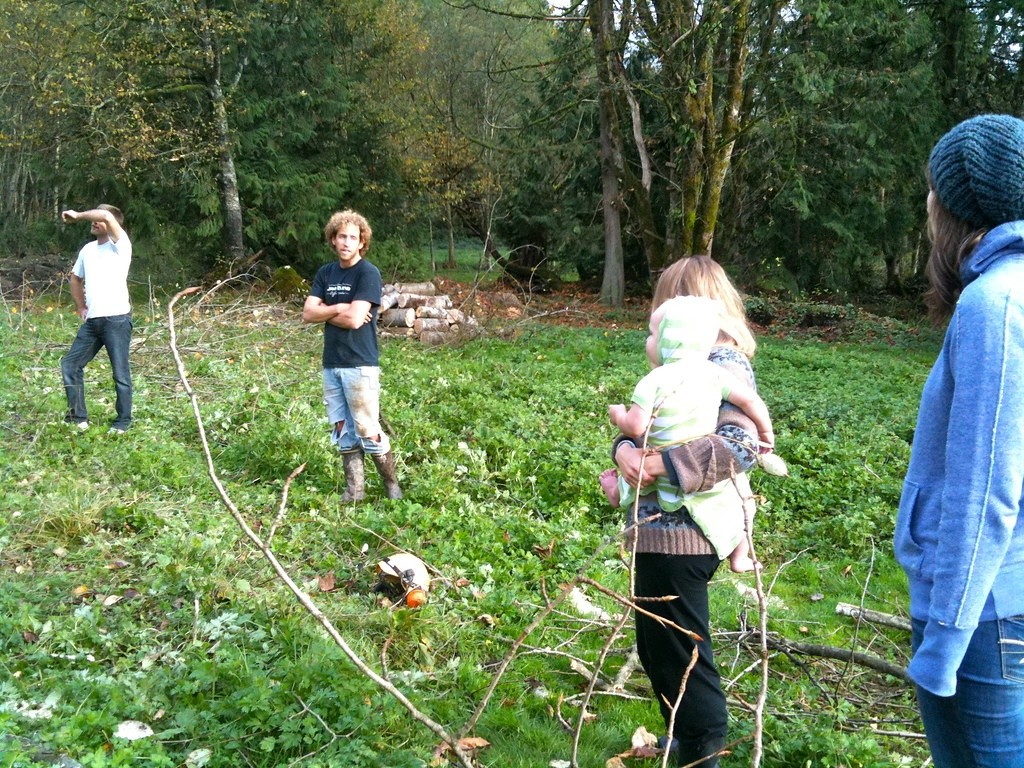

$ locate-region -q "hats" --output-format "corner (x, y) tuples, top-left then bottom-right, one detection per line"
(930, 113), (1024, 227)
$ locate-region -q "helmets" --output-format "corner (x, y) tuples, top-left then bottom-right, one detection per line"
(378, 553), (430, 608)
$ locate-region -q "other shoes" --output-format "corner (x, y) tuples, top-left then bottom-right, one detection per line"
(106, 422), (129, 434)
(64, 415), (89, 430)
(657, 732), (681, 751)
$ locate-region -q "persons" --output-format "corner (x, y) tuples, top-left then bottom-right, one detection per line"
(61, 203), (133, 437)
(303, 210), (402, 506)
(599, 296), (774, 573)
(897, 112), (1024, 768)
(611, 254), (759, 768)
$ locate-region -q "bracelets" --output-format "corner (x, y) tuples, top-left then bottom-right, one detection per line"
(616, 439), (636, 450)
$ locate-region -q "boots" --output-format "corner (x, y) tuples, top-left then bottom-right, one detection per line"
(337, 447), (364, 503)
(371, 446), (402, 500)
